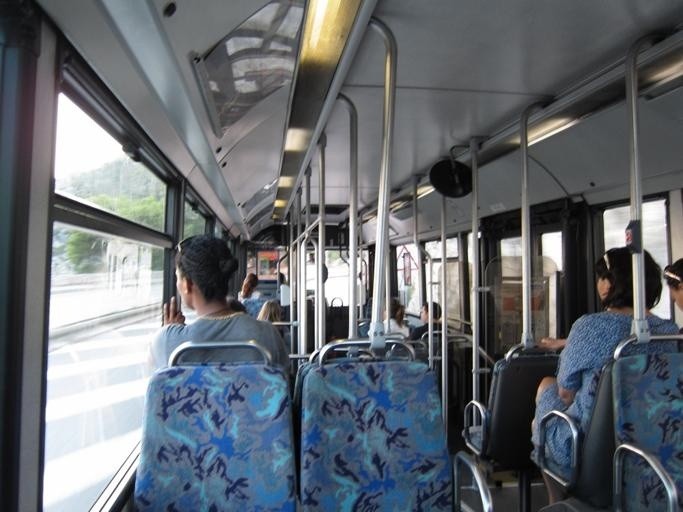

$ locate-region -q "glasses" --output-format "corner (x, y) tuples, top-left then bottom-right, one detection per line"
(174, 234), (195, 253)
(601, 247), (618, 269)
(662, 264), (682, 282)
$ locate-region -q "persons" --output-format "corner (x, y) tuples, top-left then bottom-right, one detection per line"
(151, 236), (286, 373)
(531, 247), (682, 506)
(367, 298), (442, 349)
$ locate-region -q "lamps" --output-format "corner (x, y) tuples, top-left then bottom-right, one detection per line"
(267, 0), (381, 223)
(388, 29), (683, 213)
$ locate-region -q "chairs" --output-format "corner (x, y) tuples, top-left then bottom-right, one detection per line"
(133, 320), (683, 512)
